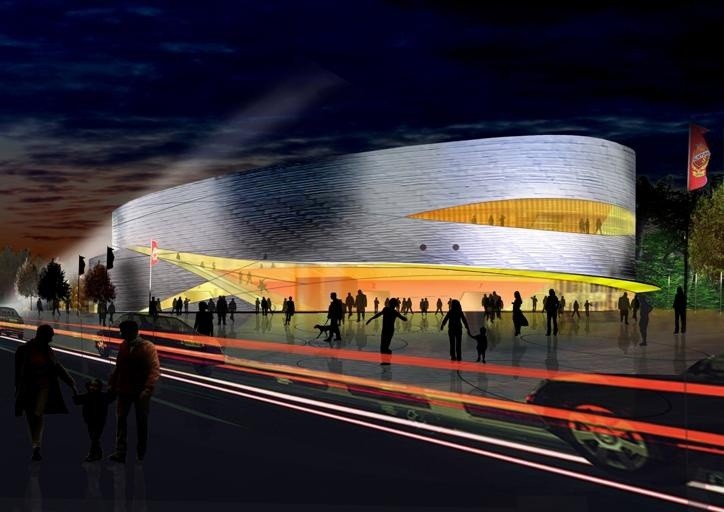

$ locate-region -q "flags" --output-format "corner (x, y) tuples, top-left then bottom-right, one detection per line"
(79, 255), (85, 275)
(151, 240), (160, 264)
(107, 247), (115, 269)
(689, 123), (711, 190)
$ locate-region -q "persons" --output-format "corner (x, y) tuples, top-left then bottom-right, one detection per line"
(177, 252), (275, 294)
(194, 296), (237, 336)
(441, 299), (470, 360)
(374, 297), (452, 314)
(256, 296), (295, 325)
(108, 320), (161, 464)
(324, 290), (367, 343)
(72, 378), (117, 461)
(617, 285), (685, 346)
(149, 296), (162, 316)
(64, 296), (71, 313)
(482, 288), (592, 337)
(98, 301), (115, 325)
(172, 297), (188, 316)
(366, 299), (407, 354)
(12, 324), (78, 448)
(465, 213), (606, 237)
(51, 297), (61, 316)
(37, 298), (41, 316)
(467, 327), (487, 364)
